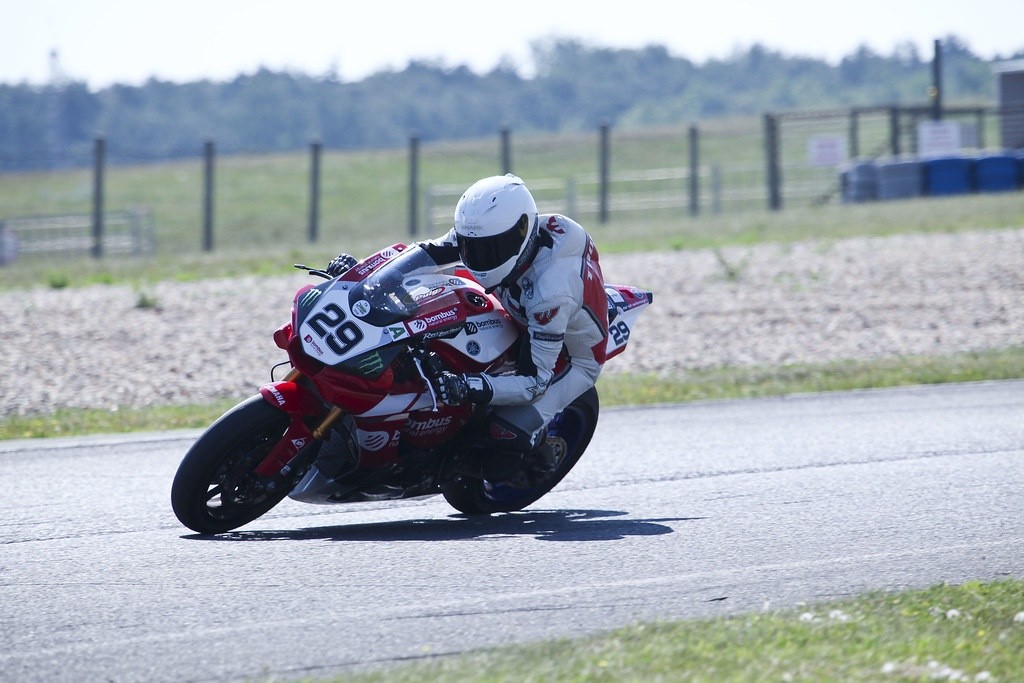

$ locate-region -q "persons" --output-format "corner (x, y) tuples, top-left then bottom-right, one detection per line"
(327, 172), (608, 501)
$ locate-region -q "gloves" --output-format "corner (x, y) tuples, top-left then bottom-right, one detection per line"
(326, 254), (358, 276)
(437, 371), (493, 406)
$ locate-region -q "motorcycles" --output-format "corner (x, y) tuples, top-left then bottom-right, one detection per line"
(169, 240), (654, 533)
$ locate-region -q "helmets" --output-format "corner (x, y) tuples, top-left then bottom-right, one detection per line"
(453, 173), (539, 290)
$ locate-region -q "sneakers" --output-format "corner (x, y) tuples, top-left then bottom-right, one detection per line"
(484, 451), (524, 484)
